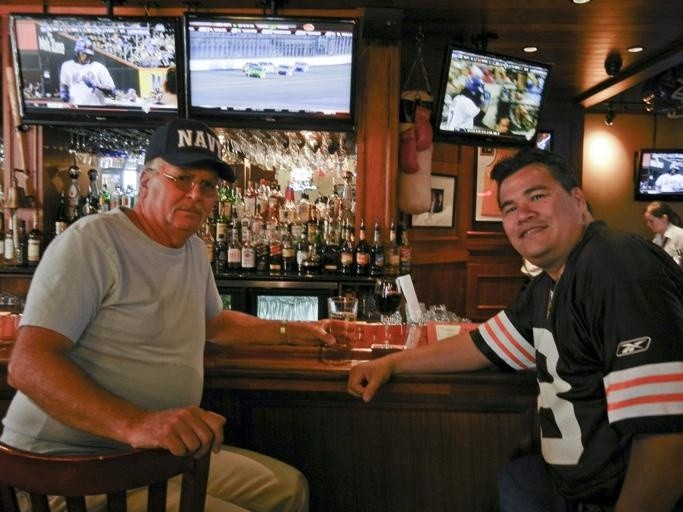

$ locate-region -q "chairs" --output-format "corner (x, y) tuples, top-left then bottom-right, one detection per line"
(0, 434), (213, 512)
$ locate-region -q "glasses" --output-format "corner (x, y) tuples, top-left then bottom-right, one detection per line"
(162, 172), (221, 196)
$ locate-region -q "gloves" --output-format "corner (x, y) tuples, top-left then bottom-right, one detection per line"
(59, 86), (69, 101)
(83, 76), (98, 89)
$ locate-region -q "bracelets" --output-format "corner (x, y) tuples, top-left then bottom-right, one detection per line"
(280, 319), (287, 346)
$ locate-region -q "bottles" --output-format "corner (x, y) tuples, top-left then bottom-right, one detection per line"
(0, 172), (134, 271)
(0, 291), (28, 345)
(199, 177), (413, 277)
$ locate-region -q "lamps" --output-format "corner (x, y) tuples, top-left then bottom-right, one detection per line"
(604, 99), (615, 127)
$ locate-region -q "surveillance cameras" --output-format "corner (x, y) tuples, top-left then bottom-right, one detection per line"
(605, 54), (622, 75)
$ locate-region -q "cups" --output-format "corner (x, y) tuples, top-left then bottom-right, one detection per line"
(327, 295), (359, 346)
(380, 301), (471, 325)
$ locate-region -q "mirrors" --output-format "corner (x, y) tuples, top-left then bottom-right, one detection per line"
(41, 125), (153, 247)
(195, 131), (355, 243)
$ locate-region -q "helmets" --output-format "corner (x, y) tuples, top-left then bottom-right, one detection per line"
(75, 38), (94, 56)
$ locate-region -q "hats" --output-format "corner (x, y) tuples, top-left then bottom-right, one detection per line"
(146, 118), (235, 183)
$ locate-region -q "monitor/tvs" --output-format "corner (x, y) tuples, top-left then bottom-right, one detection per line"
(184, 12), (359, 133)
(432, 43), (553, 150)
(9, 12), (189, 129)
(633, 148), (683, 201)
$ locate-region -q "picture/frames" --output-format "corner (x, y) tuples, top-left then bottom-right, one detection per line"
(408, 175), (458, 228)
(474, 147), (520, 222)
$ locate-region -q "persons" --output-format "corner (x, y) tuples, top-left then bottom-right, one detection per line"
(641, 155), (682, 193)
(441, 62), (545, 136)
(644, 201), (682, 264)
(2, 145), (355, 512)
(29, 22), (174, 104)
(347, 148), (682, 511)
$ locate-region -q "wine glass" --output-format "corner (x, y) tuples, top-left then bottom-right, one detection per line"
(214, 126), (356, 176)
(60, 125), (156, 170)
(372, 277), (405, 350)
